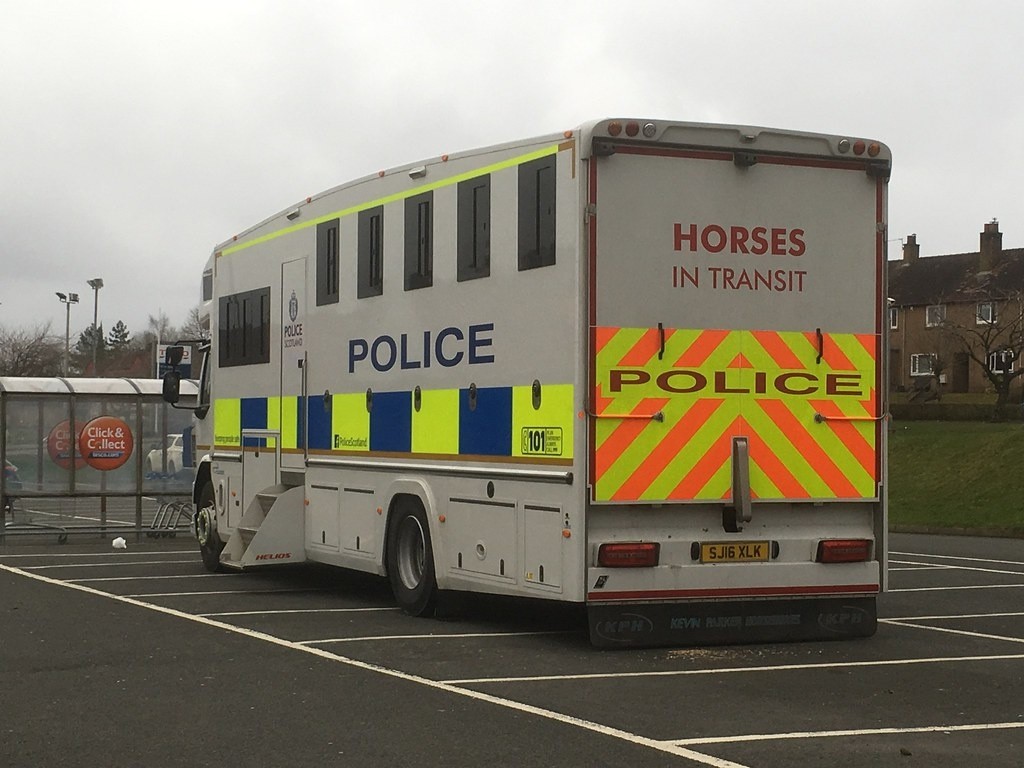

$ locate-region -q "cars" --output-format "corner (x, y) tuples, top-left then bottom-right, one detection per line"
(144, 432), (184, 476)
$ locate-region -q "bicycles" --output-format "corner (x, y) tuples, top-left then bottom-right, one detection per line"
(6, 457), (21, 520)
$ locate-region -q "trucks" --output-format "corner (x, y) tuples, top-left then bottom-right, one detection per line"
(160, 110), (896, 654)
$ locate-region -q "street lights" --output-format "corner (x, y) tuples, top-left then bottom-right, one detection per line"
(899, 305), (914, 392)
(55, 291), (80, 378)
(86, 278), (104, 378)
